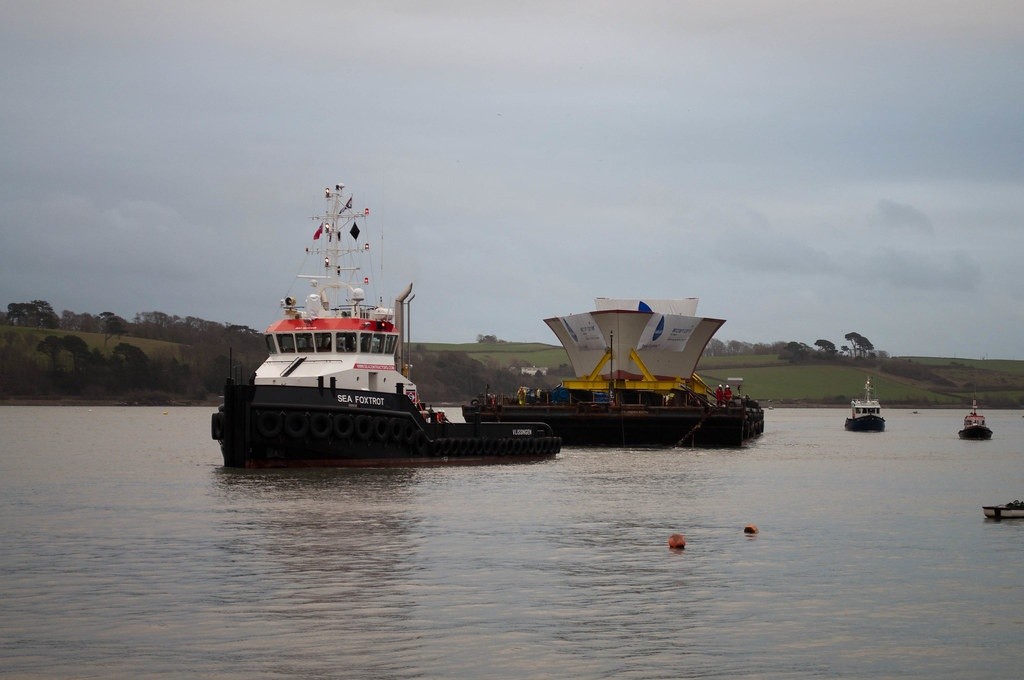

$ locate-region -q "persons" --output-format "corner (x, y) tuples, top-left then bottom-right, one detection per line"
(428, 405), (434, 412)
(716, 384), (723, 406)
(723, 385), (732, 400)
(339, 343), (346, 351)
(437, 412), (449, 423)
(517, 387), (525, 405)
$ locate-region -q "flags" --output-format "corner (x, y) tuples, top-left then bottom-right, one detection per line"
(314, 223), (323, 239)
(339, 198), (352, 213)
(350, 223), (360, 239)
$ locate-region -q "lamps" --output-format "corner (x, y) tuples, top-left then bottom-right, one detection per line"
(284, 297), (296, 305)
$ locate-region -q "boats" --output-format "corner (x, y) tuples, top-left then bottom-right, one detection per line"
(982, 500), (1024, 518)
(957, 399), (993, 440)
(843, 377), (886, 432)
(211, 181), (565, 468)
